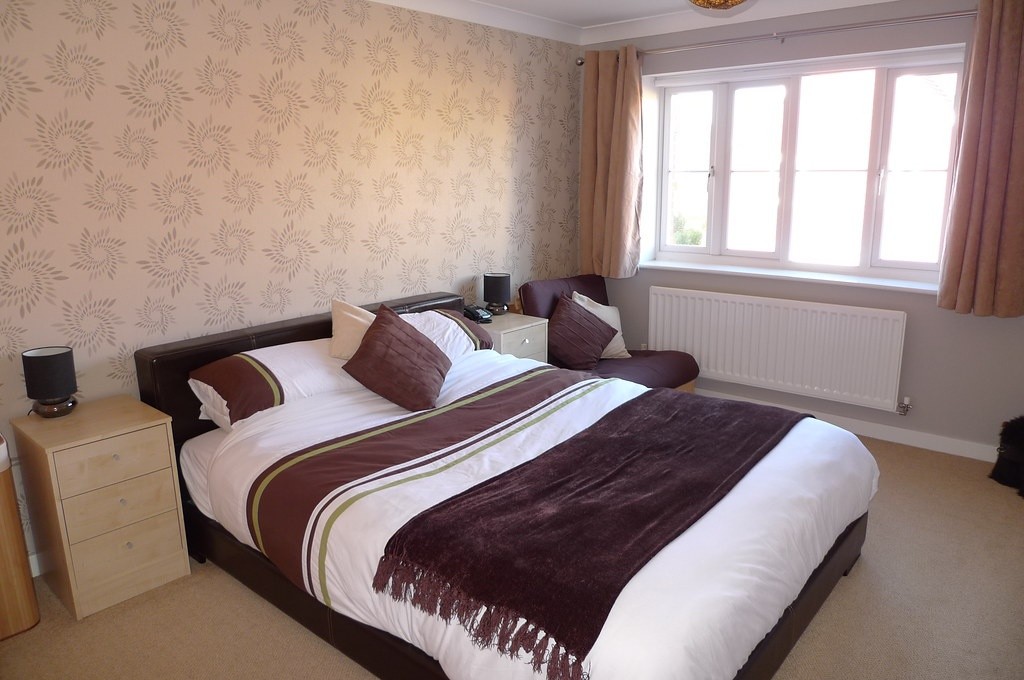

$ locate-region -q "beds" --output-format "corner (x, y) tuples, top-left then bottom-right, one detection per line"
(132, 291), (882, 680)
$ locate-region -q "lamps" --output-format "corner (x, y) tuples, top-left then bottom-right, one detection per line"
(22, 346), (80, 418)
(484, 273), (512, 316)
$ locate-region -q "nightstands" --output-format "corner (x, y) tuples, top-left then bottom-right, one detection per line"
(7, 393), (192, 620)
(475, 312), (550, 364)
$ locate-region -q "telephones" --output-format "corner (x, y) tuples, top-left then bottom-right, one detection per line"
(464, 304), (493, 320)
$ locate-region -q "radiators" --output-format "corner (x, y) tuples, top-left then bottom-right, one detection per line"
(639, 286), (912, 417)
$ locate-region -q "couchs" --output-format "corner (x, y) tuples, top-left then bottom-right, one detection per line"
(518, 274), (700, 394)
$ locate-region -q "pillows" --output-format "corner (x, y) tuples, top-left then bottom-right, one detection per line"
(329, 297), (377, 360)
(190, 309), (495, 432)
(572, 291), (633, 359)
(547, 290), (620, 370)
(339, 302), (454, 411)
